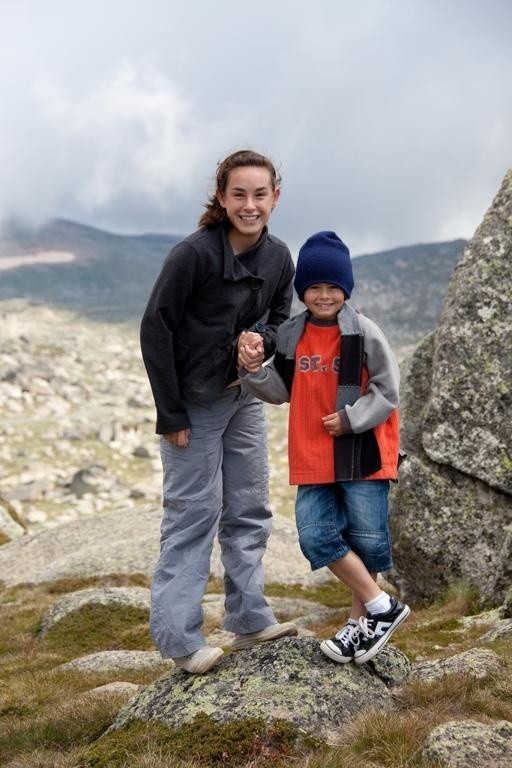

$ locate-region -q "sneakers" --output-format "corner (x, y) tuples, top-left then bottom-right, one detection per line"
(353, 595), (410, 666)
(319, 623), (361, 665)
(230, 621), (299, 653)
(172, 644), (226, 676)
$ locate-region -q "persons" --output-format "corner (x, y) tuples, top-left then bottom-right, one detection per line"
(139, 150), (298, 677)
(242, 231), (408, 666)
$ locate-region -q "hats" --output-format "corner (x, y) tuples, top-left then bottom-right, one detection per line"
(293, 231), (355, 302)
